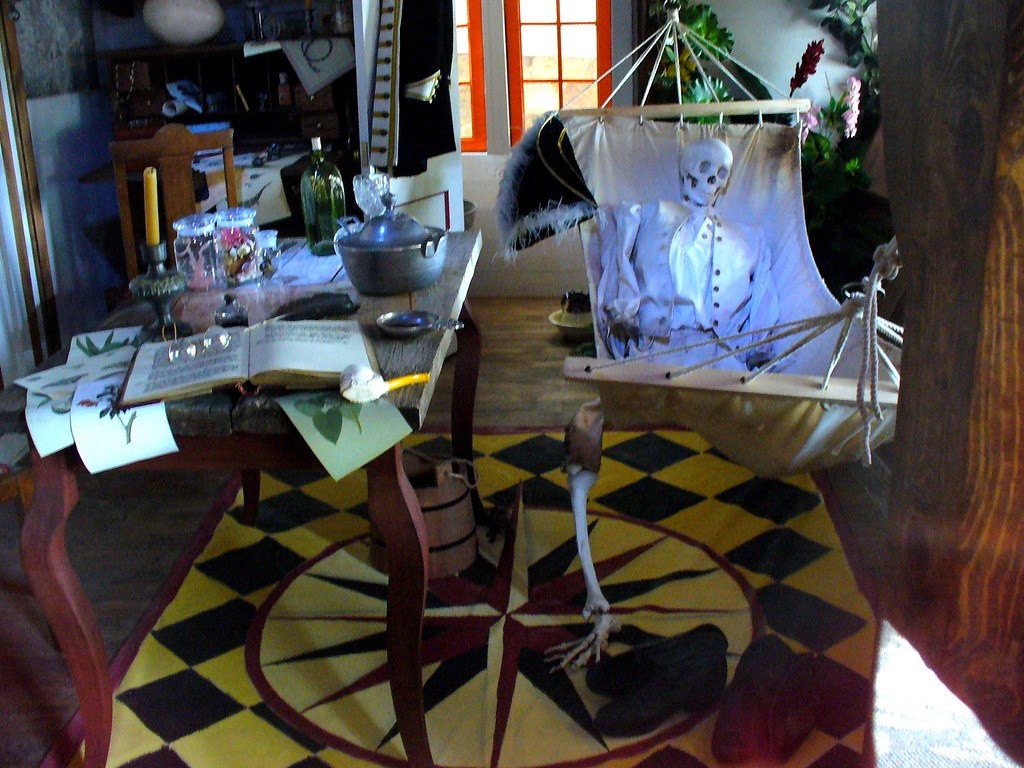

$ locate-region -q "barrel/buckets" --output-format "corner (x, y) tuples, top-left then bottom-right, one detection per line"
(369, 458), (475, 581)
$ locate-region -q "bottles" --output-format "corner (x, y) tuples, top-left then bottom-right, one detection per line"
(278, 73), (290, 104)
(300, 134), (346, 256)
(215, 294), (248, 328)
(215, 208), (258, 288)
(172, 214), (218, 289)
(257, 230), (282, 287)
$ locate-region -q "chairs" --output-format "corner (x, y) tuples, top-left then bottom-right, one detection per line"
(107, 122), (238, 281)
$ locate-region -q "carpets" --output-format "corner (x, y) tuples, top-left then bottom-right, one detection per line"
(42, 426), (880, 768)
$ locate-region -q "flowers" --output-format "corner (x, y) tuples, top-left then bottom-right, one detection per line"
(790, 37), (871, 229)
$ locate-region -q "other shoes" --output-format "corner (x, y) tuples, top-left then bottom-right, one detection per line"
(712, 633), (824, 768)
(586, 623), (729, 696)
(594, 656), (727, 738)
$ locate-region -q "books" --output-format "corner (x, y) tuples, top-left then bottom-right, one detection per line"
(117, 321), (384, 411)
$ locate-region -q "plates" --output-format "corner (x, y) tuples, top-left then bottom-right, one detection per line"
(376, 311), (465, 336)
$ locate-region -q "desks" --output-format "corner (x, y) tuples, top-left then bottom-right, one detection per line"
(0, 229), (484, 768)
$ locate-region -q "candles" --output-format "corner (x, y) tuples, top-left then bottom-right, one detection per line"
(143, 165), (160, 245)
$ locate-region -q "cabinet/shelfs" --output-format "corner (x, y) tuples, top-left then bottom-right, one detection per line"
(86, 38), (354, 158)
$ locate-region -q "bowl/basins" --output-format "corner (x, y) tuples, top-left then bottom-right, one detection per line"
(463, 198), (477, 228)
(338, 194), (447, 296)
(549, 310), (594, 338)
